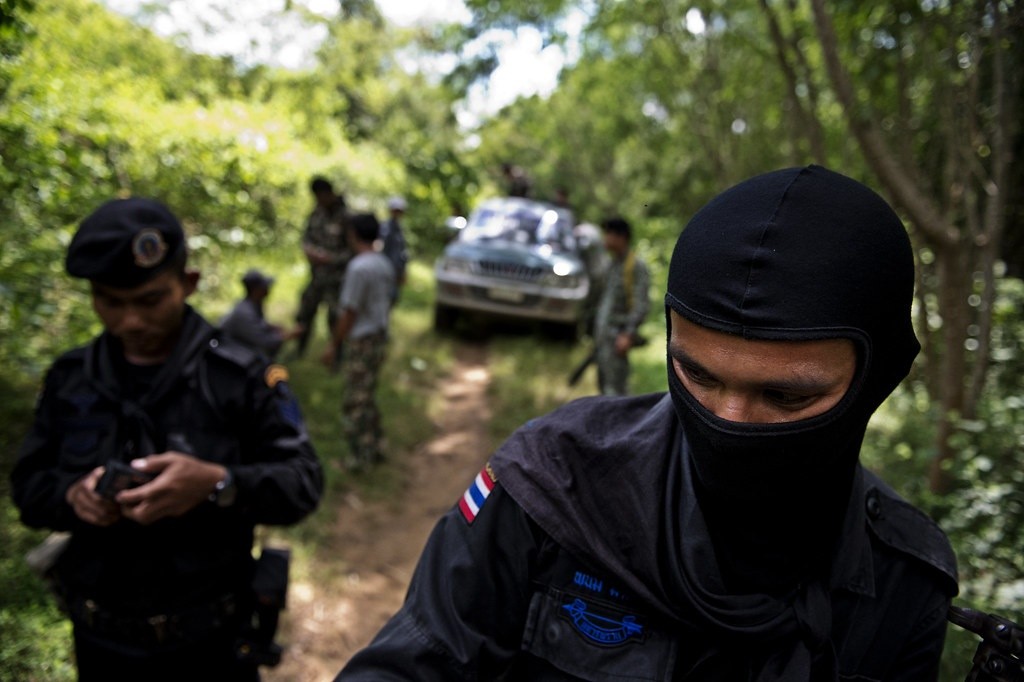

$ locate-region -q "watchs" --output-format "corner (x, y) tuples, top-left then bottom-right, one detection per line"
(211, 474), (235, 506)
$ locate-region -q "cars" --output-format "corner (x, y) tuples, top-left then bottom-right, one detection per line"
(433, 195), (592, 341)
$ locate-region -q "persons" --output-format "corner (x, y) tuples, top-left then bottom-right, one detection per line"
(585, 215), (649, 398)
(9, 197), (324, 682)
(501, 163), (568, 206)
(333, 163), (958, 681)
(219, 177), (410, 476)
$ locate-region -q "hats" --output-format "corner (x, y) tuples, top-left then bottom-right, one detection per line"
(66, 200), (187, 287)
(243, 271), (272, 287)
